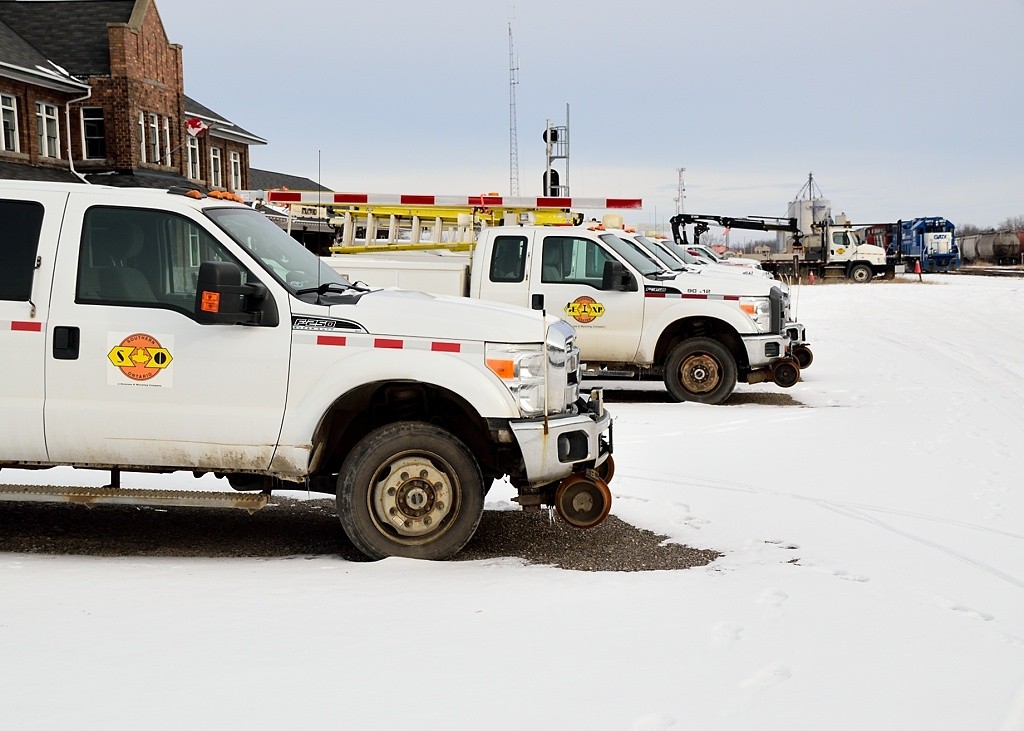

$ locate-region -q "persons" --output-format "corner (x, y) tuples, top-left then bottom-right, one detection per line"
(828, 216), (833, 225)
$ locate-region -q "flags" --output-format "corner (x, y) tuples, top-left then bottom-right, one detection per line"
(184, 118), (208, 138)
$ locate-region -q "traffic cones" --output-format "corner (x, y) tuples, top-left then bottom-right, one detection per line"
(914, 260), (922, 274)
(808, 271), (815, 284)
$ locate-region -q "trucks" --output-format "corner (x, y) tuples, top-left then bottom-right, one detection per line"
(670, 214), (889, 283)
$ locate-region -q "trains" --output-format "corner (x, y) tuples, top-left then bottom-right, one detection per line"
(861, 217), (961, 272)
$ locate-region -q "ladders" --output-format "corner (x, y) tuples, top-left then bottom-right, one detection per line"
(329, 203), (573, 253)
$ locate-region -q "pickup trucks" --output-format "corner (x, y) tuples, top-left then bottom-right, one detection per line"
(255, 223), (813, 404)
(0, 160), (616, 561)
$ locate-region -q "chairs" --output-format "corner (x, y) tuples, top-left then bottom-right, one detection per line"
(542, 242), (562, 281)
(98, 220), (160, 303)
